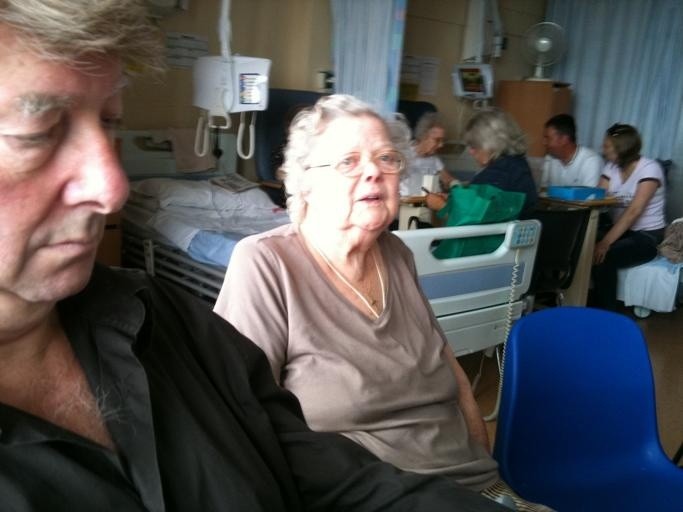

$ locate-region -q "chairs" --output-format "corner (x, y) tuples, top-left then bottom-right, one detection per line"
(491, 307), (682, 511)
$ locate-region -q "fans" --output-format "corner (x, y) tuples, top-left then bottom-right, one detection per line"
(518, 21), (567, 84)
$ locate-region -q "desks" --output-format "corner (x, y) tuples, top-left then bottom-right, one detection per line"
(537, 195), (632, 308)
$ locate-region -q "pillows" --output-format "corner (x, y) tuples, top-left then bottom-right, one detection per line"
(121, 174), (277, 215)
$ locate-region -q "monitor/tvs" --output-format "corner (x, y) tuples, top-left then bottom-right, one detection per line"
(453, 63), (492, 98)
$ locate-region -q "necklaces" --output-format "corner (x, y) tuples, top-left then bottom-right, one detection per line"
(356, 280), (381, 311)
(311, 240), (387, 318)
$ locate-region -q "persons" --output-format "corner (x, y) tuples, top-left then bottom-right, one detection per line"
(567, 121), (670, 309)
(2, 0), (519, 512)
(425, 103), (544, 295)
(206, 94), (553, 510)
(389, 110), (469, 246)
(536, 111), (610, 305)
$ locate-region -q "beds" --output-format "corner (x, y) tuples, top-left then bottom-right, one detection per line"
(109, 174), (540, 396)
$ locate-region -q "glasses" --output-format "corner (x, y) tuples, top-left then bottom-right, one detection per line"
(302, 149), (408, 177)
